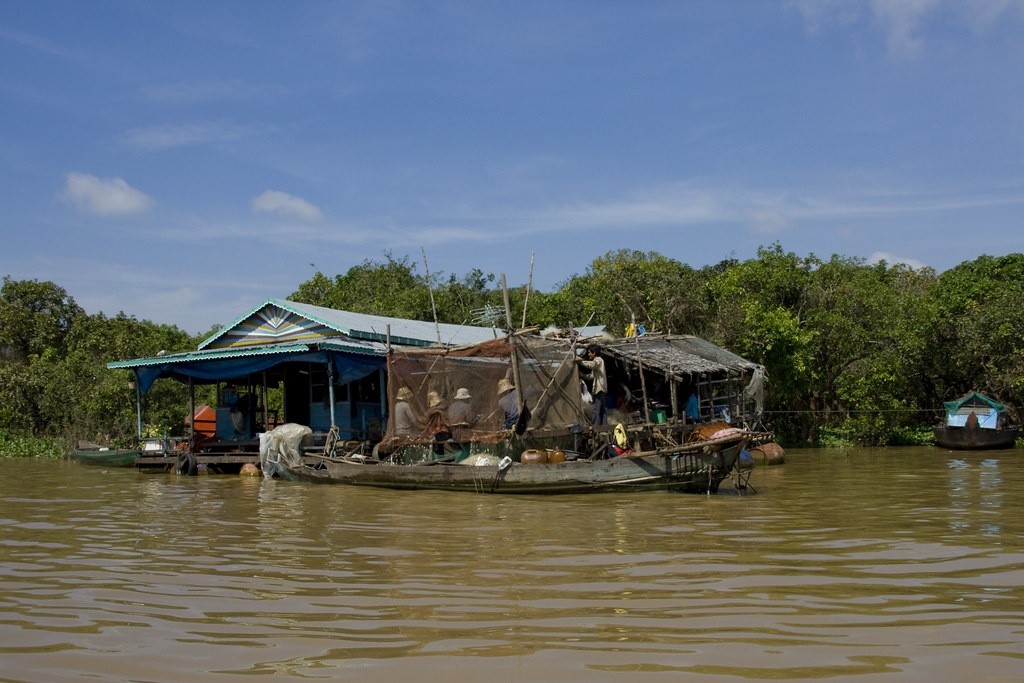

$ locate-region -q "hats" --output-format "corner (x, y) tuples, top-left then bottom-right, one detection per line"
(454, 388), (471, 400)
(497, 379), (515, 395)
(396, 388), (413, 400)
(427, 391), (443, 407)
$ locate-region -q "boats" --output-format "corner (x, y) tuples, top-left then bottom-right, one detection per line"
(268, 246), (778, 497)
(930, 390), (1020, 450)
(74, 439), (138, 467)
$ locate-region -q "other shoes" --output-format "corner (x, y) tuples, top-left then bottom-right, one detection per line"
(591, 421), (609, 426)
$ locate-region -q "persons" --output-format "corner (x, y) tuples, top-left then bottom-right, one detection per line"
(497, 378), (520, 430)
(449, 388), (481, 434)
(681, 388), (699, 419)
(427, 391), (449, 434)
(394, 386), (425, 437)
(576, 344), (607, 431)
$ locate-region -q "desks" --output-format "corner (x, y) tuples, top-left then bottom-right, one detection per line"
(199, 440), (260, 451)
